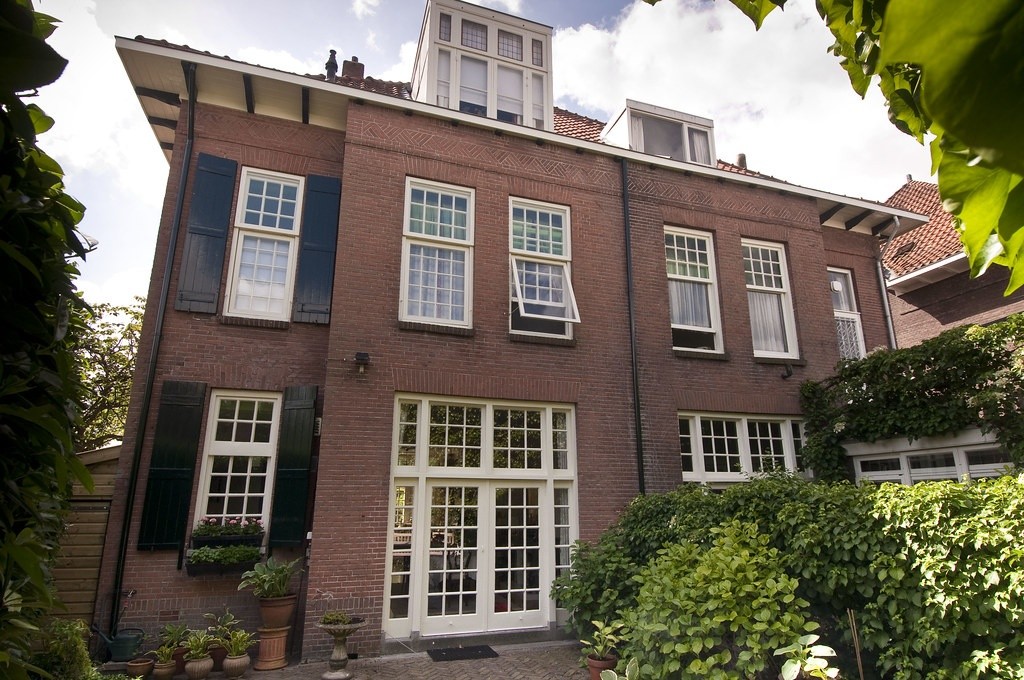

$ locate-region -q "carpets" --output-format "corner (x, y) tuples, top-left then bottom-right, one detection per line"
(427, 644), (499, 662)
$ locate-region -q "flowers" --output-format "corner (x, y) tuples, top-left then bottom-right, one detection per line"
(194, 517), (264, 534)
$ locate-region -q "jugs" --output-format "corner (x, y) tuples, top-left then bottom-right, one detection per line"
(92, 625), (145, 661)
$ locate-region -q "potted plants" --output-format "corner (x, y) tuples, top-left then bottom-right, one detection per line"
(314, 608), (367, 680)
(144, 641), (179, 680)
(158, 623), (192, 675)
(179, 630), (221, 680)
(579, 620), (624, 680)
(237, 555), (308, 628)
(219, 628), (261, 680)
(202, 607), (245, 671)
(184, 545), (263, 576)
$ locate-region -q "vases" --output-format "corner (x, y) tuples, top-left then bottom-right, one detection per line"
(192, 534), (265, 548)
(127, 659), (155, 678)
(255, 625), (291, 670)
